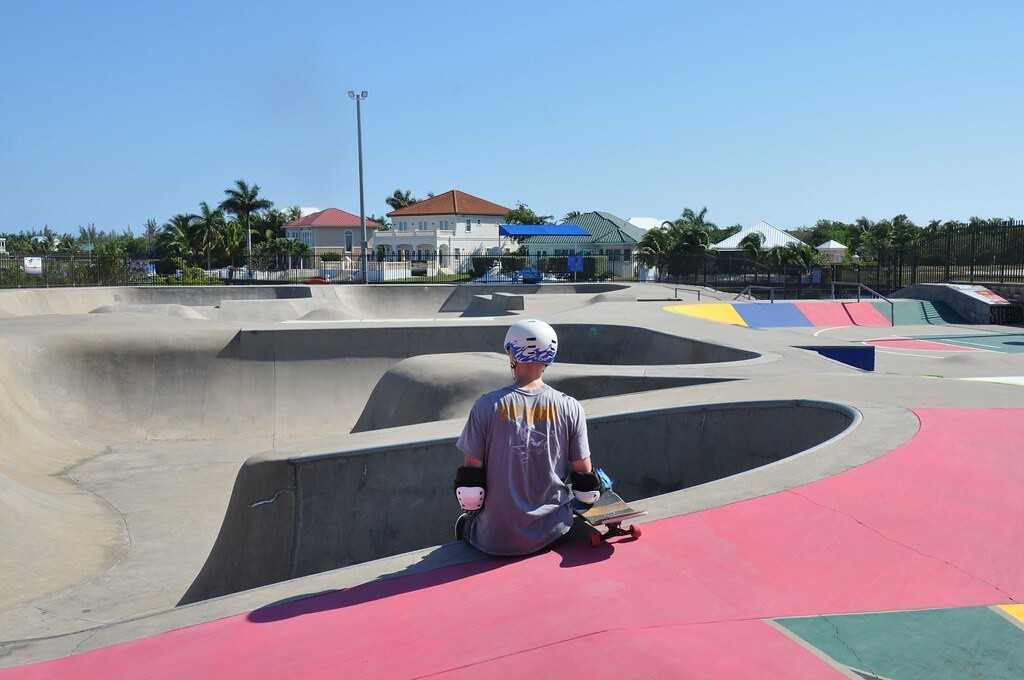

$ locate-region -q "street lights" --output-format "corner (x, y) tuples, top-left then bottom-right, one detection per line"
(346, 89), (368, 286)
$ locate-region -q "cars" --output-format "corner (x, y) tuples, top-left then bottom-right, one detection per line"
(300, 274), (327, 284)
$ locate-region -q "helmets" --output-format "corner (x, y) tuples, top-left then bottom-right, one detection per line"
(504, 319), (558, 365)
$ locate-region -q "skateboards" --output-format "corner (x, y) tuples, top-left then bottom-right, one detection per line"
(573, 488), (648, 547)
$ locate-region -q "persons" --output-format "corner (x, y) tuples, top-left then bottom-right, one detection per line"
(454, 319), (600, 557)
